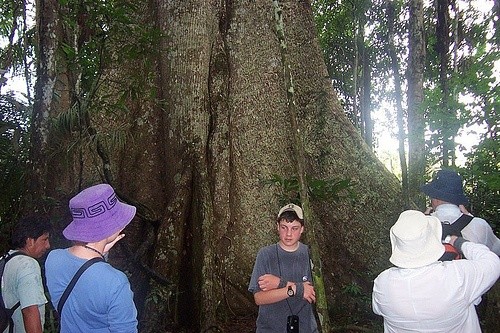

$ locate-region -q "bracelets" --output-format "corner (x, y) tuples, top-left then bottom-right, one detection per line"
(278, 277), (288, 288)
(295, 282), (304, 297)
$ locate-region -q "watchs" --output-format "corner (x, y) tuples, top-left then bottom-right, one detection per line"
(287, 286), (294, 297)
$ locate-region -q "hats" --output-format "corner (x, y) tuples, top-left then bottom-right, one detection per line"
(388, 210), (445, 270)
(62, 184), (137, 242)
(276, 203), (303, 220)
(421, 169), (468, 206)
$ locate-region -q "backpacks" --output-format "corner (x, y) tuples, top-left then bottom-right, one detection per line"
(0, 251), (46, 333)
(424, 214), (466, 261)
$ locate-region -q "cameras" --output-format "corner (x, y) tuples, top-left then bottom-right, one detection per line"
(287, 315), (300, 333)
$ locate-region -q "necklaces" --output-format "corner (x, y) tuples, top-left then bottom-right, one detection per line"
(84, 245), (105, 260)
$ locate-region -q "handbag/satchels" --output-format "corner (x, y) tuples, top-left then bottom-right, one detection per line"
(44, 301), (61, 333)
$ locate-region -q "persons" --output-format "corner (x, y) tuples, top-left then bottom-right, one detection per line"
(45, 184), (139, 333)
(0, 215), (52, 333)
(421, 170), (500, 305)
(372, 210), (500, 333)
(248, 204), (319, 333)
